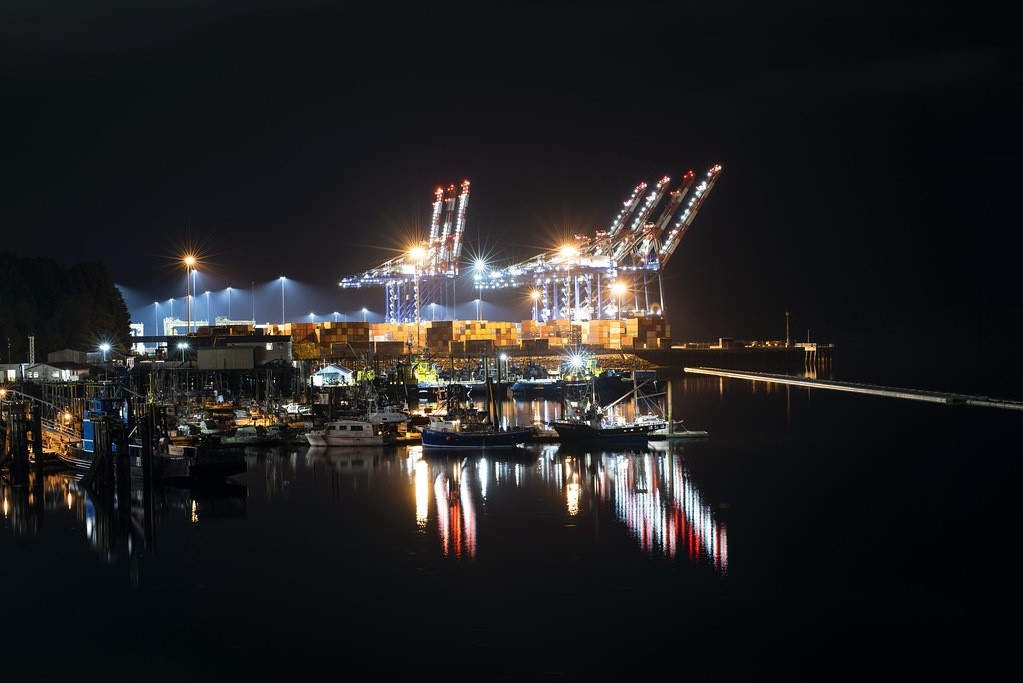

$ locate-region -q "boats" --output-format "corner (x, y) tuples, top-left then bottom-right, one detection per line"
(602, 370), (684, 435)
(54, 358), (487, 478)
(422, 356), (538, 448)
(548, 366), (648, 449)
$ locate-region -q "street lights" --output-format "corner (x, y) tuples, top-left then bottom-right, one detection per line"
(473, 257), (487, 319)
(278, 276), (290, 324)
(182, 254), (198, 332)
(100, 342), (112, 360)
(177, 340), (189, 363)
